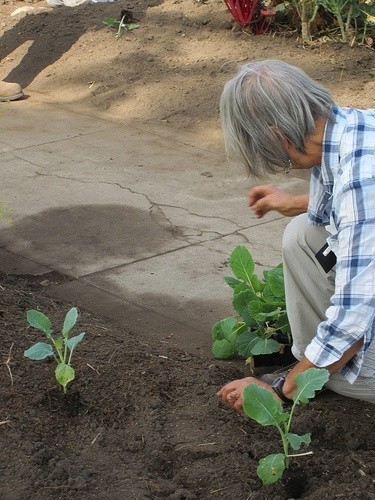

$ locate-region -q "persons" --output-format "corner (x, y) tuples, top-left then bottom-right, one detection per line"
(215, 60), (374, 413)
(0, 79), (25, 101)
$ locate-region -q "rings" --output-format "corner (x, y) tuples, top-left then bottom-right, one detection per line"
(227, 391), (239, 401)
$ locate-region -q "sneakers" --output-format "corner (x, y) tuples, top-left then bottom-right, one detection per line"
(0, 80), (24, 101)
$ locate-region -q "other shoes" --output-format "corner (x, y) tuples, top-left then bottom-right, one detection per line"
(260, 360), (299, 386)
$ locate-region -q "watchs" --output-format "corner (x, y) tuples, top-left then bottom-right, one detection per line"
(271, 377), (295, 406)
